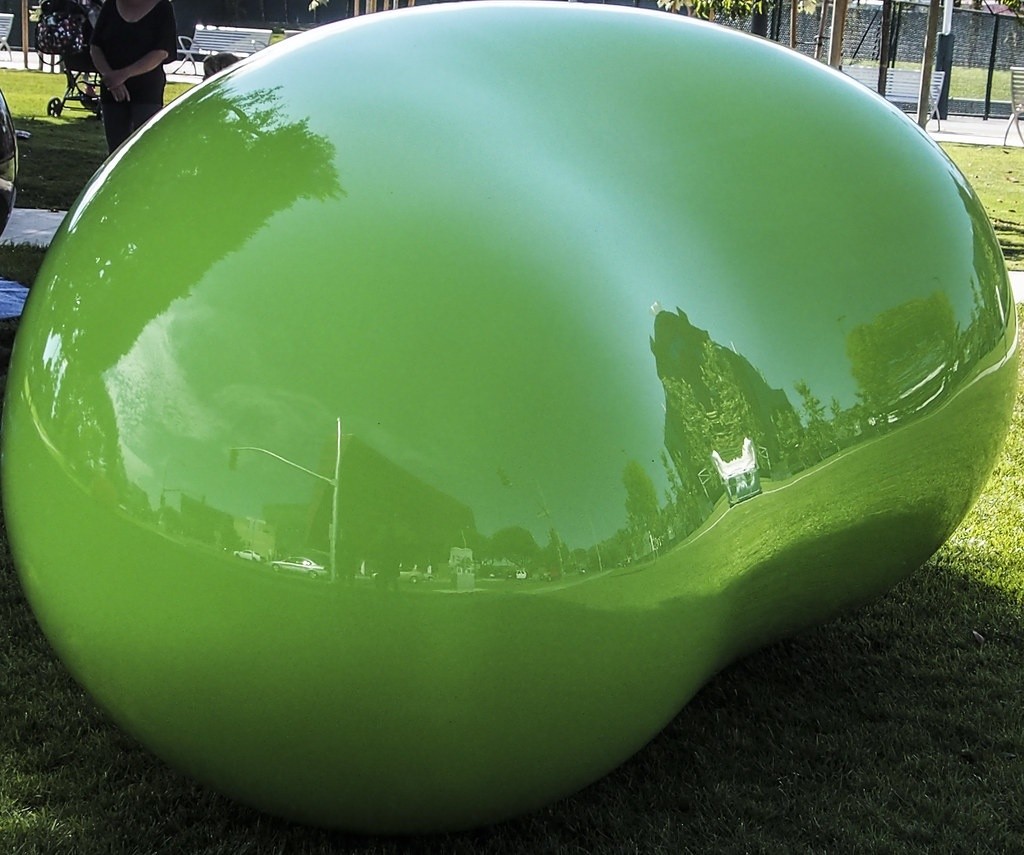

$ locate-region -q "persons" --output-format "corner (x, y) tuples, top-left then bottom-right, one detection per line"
(88, 1), (103, 29)
(202, 53), (238, 82)
(90, 0), (178, 156)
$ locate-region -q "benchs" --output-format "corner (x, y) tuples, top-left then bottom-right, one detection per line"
(839, 65), (945, 132)
(173, 24), (273, 76)
(1004, 67), (1024, 146)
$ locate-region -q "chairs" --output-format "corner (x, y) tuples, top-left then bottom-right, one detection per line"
(0, 14), (14, 60)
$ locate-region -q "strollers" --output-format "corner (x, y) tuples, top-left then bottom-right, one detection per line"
(34, 0), (104, 121)
